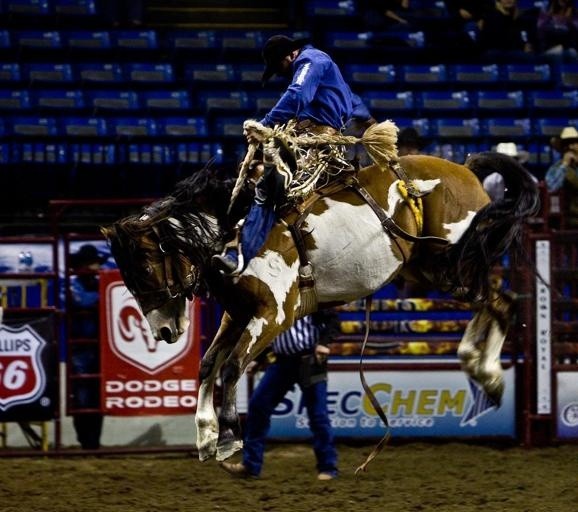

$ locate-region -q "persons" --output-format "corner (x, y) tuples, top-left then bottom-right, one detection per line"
(384, 0), (578, 55)
(543, 126), (578, 228)
(64, 243), (110, 448)
(210, 27), (380, 286)
(220, 309), (341, 483)
(496, 141), (530, 166)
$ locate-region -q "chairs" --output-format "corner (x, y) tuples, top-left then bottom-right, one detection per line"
(0, 0), (578, 217)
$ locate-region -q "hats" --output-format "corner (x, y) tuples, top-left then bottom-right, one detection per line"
(498, 142), (529, 163)
(69, 245), (108, 268)
(398, 128), (432, 150)
(262, 35), (309, 82)
(550, 127), (578, 152)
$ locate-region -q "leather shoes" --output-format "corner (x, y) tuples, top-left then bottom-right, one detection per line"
(318, 472), (336, 479)
(212, 255), (240, 285)
(221, 462), (257, 478)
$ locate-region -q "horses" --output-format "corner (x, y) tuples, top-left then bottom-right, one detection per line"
(98, 151), (541, 462)
